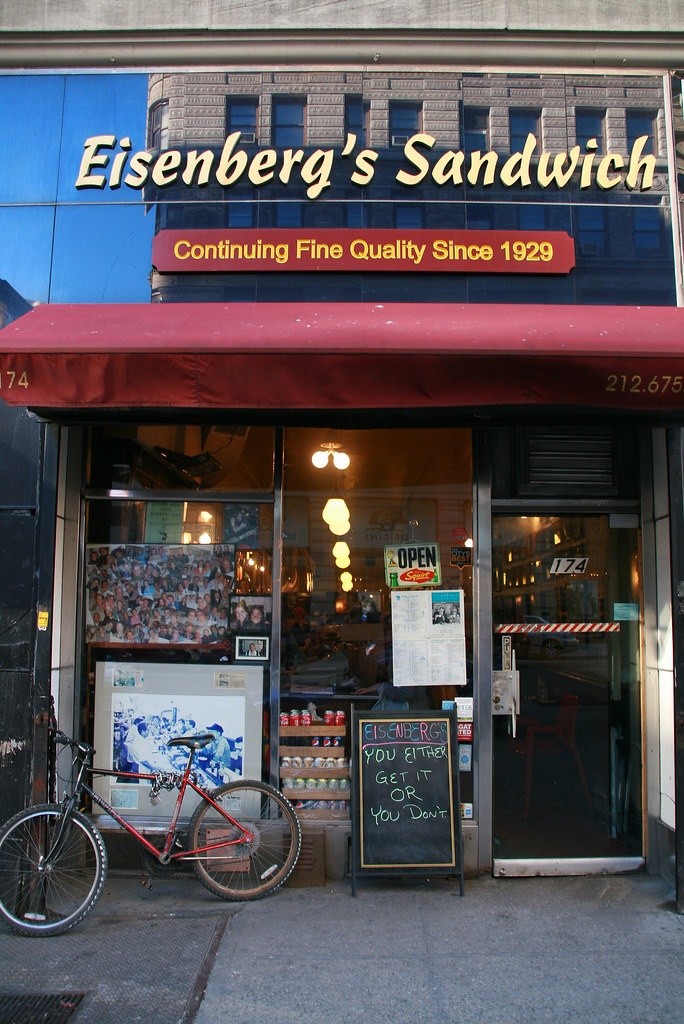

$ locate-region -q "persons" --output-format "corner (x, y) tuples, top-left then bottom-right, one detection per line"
(280, 588), (450, 714)
(86, 541), (269, 660)
(114, 706), (242, 784)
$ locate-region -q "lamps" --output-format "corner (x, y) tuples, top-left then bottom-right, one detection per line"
(312, 428), (350, 470)
(322, 481), (354, 593)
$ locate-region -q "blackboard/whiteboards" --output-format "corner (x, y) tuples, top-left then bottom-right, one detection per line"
(350, 709), (465, 878)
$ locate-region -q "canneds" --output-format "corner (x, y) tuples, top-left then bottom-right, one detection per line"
(279, 709), (349, 810)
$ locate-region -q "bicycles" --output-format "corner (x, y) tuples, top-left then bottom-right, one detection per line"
(0, 725), (303, 937)
